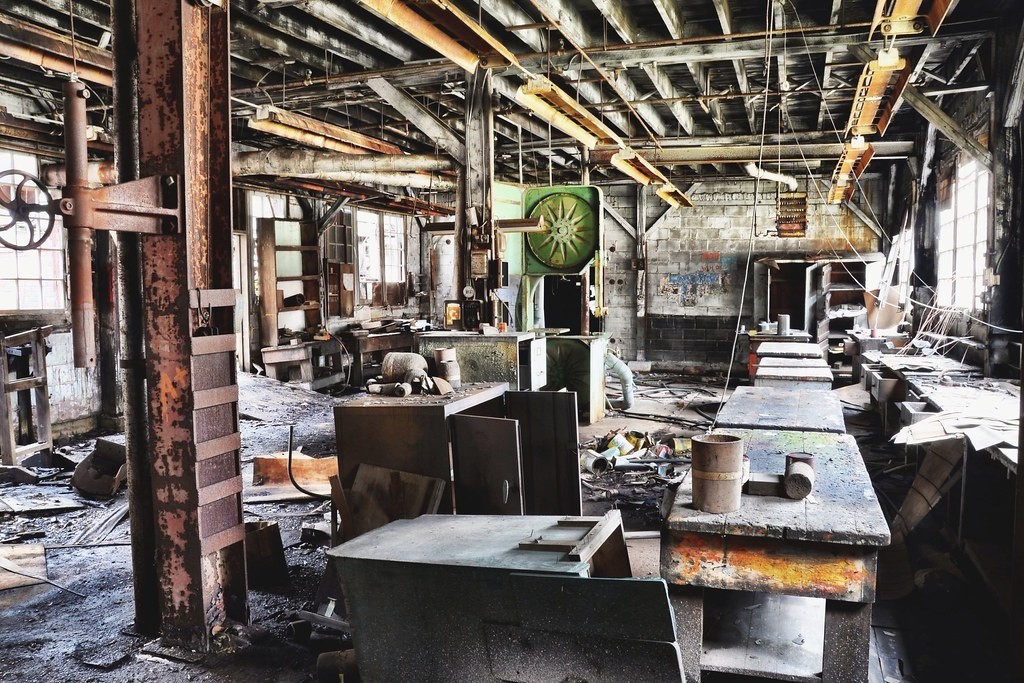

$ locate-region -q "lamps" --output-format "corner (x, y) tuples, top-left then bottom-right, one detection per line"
(423, 214), (546, 236)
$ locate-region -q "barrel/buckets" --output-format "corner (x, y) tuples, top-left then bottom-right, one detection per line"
(580, 449), (608, 475)
(668, 438), (692, 453)
(607, 433), (635, 456)
(627, 431), (645, 452)
(691, 434), (743, 514)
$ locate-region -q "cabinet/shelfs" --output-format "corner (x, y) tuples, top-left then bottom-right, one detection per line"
(333, 383), (583, 516)
(767, 259), (886, 374)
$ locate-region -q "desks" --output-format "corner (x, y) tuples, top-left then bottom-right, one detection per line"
(335, 331), (413, 387)
(660, 428), (891, 683)
(715, 386), (846, 434)
(747, 333), (812, 354)
(260, 341), (345, 392)
(756, 342), (823, 358)
(754, 357), (834, 389)
(843, 329), (1021, 476)
